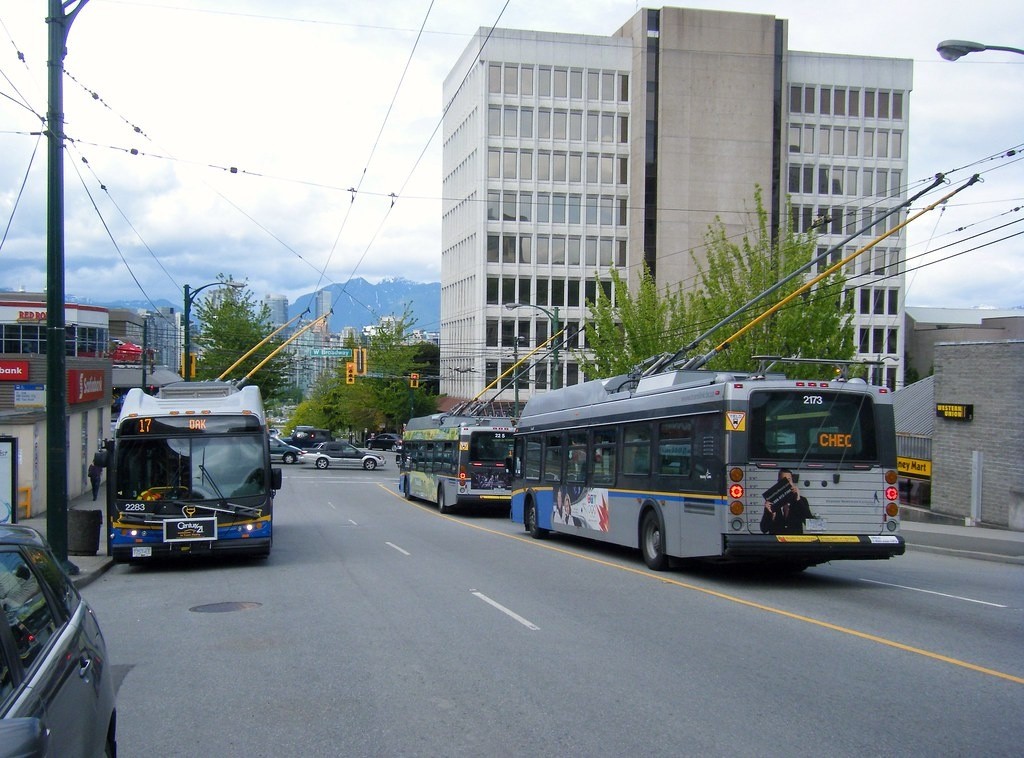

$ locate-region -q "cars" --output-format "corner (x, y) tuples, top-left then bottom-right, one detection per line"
(300, 440), (386, 471)
(268, 423), (335, 464)
(364, 433), (402, 452)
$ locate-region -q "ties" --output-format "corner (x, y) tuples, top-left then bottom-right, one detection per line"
(783, 503), (788, 520)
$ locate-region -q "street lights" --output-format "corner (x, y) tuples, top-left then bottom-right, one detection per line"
(182, 280), (245, 381)
(504, 301), (560, 389)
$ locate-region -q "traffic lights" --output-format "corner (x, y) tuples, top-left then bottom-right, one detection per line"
(346, 361), (354, 384)
(410, 373), (418, 388)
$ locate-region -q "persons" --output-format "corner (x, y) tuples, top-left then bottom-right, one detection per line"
(760, 468), (811, 535)
(90, 460), (102, 501)
(568, 438), (586, 480)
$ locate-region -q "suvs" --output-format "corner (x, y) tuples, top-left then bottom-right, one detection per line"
(1, 523), (119, 758)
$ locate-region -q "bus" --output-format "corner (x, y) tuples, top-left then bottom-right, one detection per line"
(510, 174), (983, 573)
(397, 322), (586, 516)
(89, 305), (336, 570)
